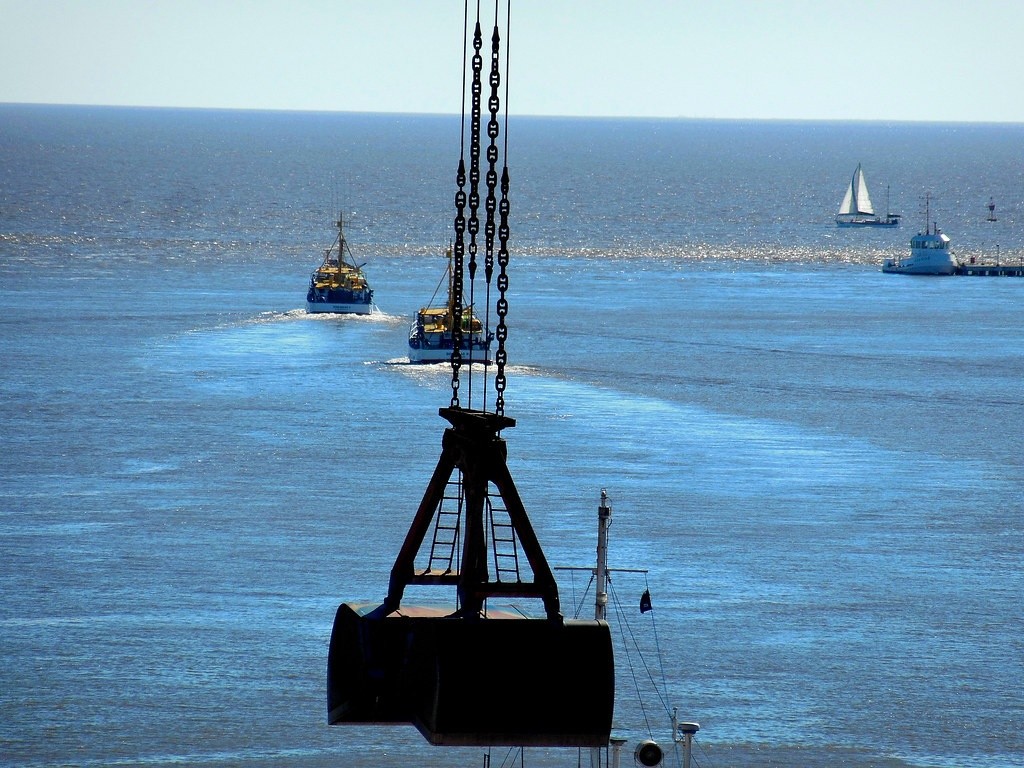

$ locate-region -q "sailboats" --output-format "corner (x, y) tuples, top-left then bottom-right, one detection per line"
(987, 197), (998, 221)
(834, 163), (900, 228)
(305, 171), (373, 315)
(408, 191), (495, 365)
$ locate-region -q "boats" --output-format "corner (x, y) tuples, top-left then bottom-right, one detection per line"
(883, 192), (958, 276)
